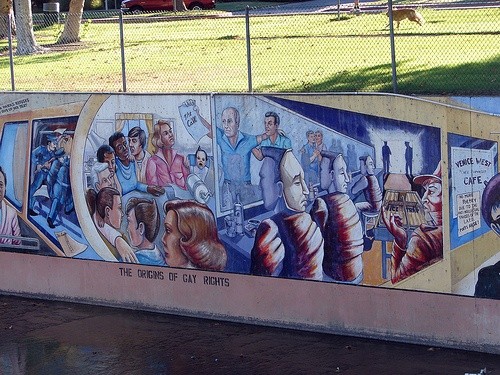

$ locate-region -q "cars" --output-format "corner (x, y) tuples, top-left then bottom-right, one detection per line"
(121, 0), (216, 14)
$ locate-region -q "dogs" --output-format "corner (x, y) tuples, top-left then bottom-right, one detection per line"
(384, 5), (422, 28)
